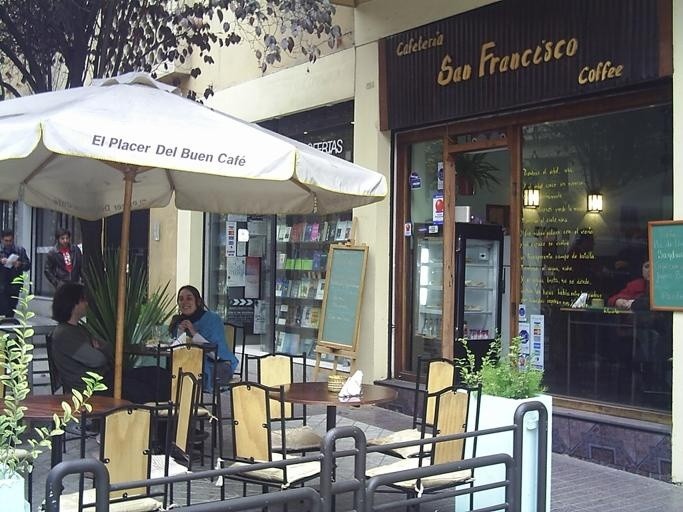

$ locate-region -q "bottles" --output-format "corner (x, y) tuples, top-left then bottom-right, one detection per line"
(464, 322), (489, 339)
(421, 318), (441, 337)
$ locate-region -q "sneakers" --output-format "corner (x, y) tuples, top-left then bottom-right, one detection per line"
(189, 429), (209, 442)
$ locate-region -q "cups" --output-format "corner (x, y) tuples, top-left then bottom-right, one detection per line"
(152, 326), (170, 344)
(592, 299), (604, 307)
(327, 376), (348, 393)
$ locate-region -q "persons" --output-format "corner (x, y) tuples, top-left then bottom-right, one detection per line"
(1, 228), (31, 317)
(160, 284), (236, 418)
(544, 229), (660, 391)
(49, 279), (210, 444)
(43, 228), (83, 294)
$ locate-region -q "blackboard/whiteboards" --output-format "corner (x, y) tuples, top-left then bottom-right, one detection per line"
(317, 244), (369, 352)
(648, 219), (683, 312)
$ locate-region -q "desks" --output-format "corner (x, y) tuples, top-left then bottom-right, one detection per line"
(559, 307), (673, 405)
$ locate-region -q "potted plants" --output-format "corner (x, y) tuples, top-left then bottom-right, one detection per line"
(455, 327), (552, 512)
(456, 152), (502, 196)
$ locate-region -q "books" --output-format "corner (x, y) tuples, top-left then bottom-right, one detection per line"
(271, 212), (352, 367)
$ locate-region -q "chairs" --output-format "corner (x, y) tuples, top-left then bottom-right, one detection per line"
(0, 318), (482, 511)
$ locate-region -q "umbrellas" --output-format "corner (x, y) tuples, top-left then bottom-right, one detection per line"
(0, 64), (385, 407)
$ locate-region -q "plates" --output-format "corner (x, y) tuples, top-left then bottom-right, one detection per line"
(588, 306), (606, 309)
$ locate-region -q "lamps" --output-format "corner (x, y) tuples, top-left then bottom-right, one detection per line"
(523, 184), (539, 209)
(587, 192), (603, 214)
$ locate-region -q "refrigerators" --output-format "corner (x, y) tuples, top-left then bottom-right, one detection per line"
(502, 236), (542, 354)
